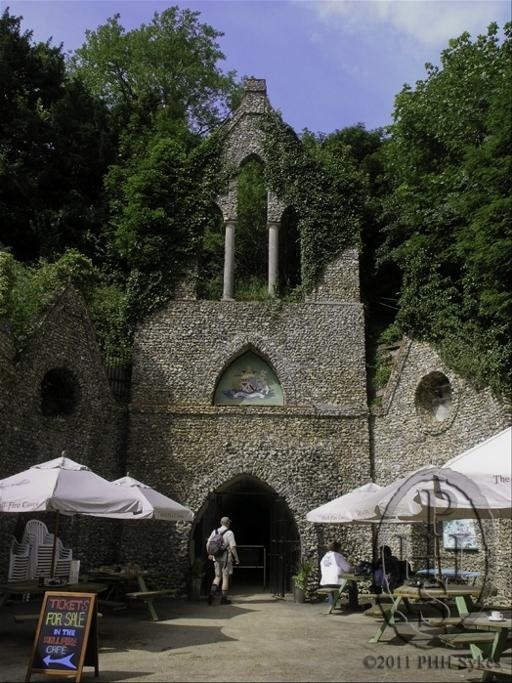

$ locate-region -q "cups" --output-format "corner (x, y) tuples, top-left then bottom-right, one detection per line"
(492, 611), (504, 619)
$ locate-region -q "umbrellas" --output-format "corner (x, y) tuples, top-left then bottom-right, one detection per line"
(78, 471), (195, 565)
(305, 427), (512, 578)
(0, 450), (142, 578)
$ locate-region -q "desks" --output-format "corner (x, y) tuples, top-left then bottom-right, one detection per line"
(0, 568), (160, 625)
(327, 572), (512, 683)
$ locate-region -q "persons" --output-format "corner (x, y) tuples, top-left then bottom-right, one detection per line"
(319, 542), (358, 610)
(374, 546), (398, 603)
(206, 516), (240, 605)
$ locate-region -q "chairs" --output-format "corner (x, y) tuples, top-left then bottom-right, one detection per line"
(0, 519), (80, 586)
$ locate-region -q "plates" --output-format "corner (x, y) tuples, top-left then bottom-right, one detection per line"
(488, 618), (506, 621)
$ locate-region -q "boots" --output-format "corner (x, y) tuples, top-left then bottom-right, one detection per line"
(220, 596), (231, 604)
(208, 594), (213, 605)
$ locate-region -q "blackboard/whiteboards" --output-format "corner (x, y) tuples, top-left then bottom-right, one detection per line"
(27, 590), (97, 675)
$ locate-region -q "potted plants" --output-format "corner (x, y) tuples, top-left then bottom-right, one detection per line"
(288, 555), (316, 603)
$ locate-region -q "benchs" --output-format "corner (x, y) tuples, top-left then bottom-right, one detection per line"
(125, 588), (177, 622)
(317, 588), (393, 640)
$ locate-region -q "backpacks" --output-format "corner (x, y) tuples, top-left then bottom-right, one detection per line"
(208, 528), (230, 555)
